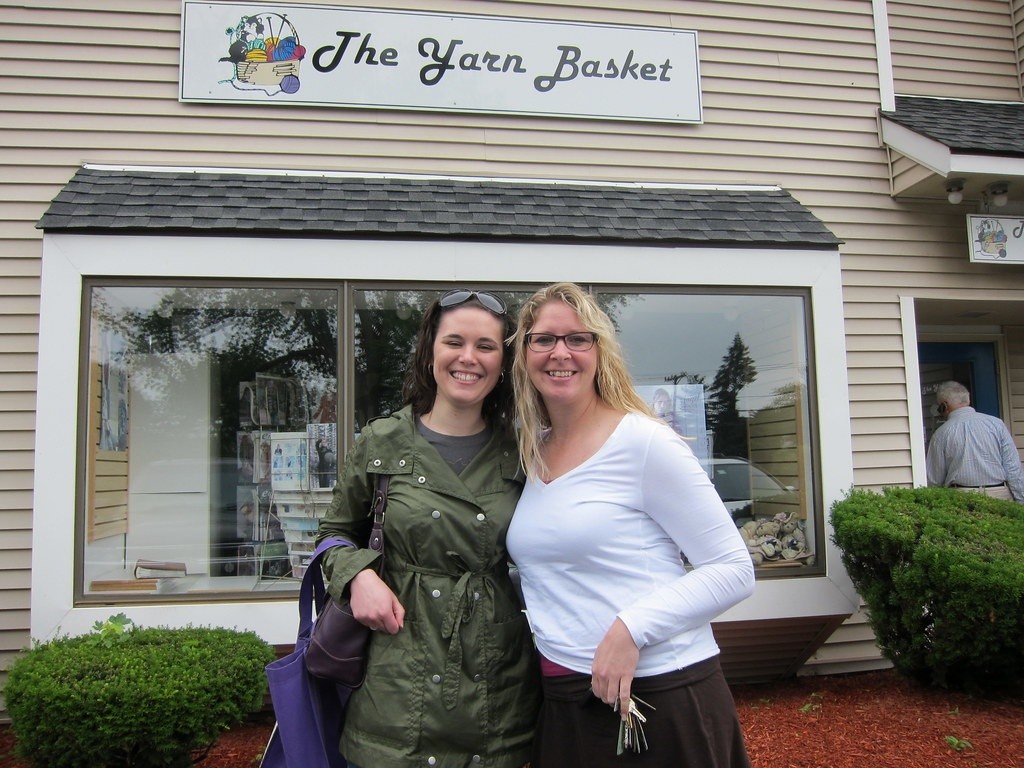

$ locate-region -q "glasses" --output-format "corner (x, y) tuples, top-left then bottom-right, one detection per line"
(524, 331), (599, 353)
(937, 401), (947, 413)
(436, 288), (510, 338)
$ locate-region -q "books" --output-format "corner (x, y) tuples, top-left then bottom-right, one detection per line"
(134, 559), (187, 579)
(235, 372), (362, 579)
(88, 576), (161, 591)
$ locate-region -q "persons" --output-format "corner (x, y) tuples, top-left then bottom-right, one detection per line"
(652, 388), (682, 434)
(318, 287), (542, 768)
(503, 282), (756, 768)
(273, 444), (282, 467)
(315, 439), (333, 487)
(925, 381), (1024, 502)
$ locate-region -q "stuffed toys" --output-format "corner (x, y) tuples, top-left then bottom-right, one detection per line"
(738, 512), (808, 565)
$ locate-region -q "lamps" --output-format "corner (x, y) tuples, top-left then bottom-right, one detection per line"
(986, 180), (1011, 209)
(943, 178), (967, 204)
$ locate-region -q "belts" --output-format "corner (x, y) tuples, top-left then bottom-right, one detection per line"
(950, 481), (1008, 488)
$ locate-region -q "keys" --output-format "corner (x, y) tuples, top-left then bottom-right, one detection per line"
(623, 697), (657, 753)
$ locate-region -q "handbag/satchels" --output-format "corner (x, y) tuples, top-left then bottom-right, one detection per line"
(303, 473), (390, 691)
(257, 539), (358, 768)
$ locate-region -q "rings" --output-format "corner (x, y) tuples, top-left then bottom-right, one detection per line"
(370, 627), (377, 631)
